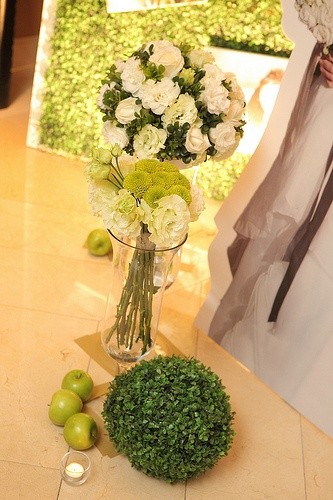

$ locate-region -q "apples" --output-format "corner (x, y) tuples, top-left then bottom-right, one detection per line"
(64, 412), (98, 451)
(62, 370), (94, 403)
(88, 230), (112, 256)
(48, 389), (84, 427)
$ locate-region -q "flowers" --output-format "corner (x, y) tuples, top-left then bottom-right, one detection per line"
(295, 0), (333, 55)
(87, 142), (206, 248)
(96, 40), (247, 171)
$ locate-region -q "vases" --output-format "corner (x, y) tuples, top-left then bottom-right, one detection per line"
(101, 227), (188, 363)
